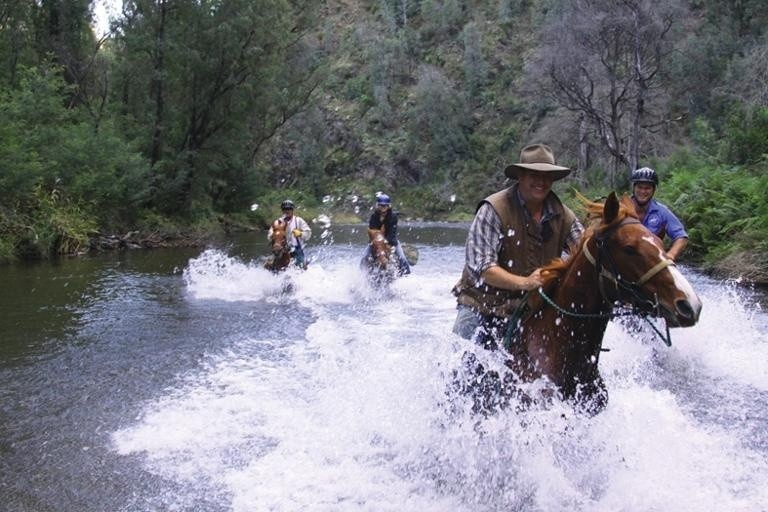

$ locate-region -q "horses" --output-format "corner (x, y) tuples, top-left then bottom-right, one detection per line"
(360, 224), (400, 290)
(264, 221), (294, 274)
(447, 188), (703, 421)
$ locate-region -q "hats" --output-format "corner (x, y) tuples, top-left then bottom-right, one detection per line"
(505, 143), (571, 181)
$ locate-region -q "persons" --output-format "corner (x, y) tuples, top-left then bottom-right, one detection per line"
(450, 144), (586, 388)
(268, 200), (312, 269)
(629, 167), (689, 262)
(361, 194), (410, 275)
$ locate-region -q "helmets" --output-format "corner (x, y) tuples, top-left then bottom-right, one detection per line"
(282, 200), (294, 210)
(632, 168), (657, 182)
(378, 195), (390, 205)
